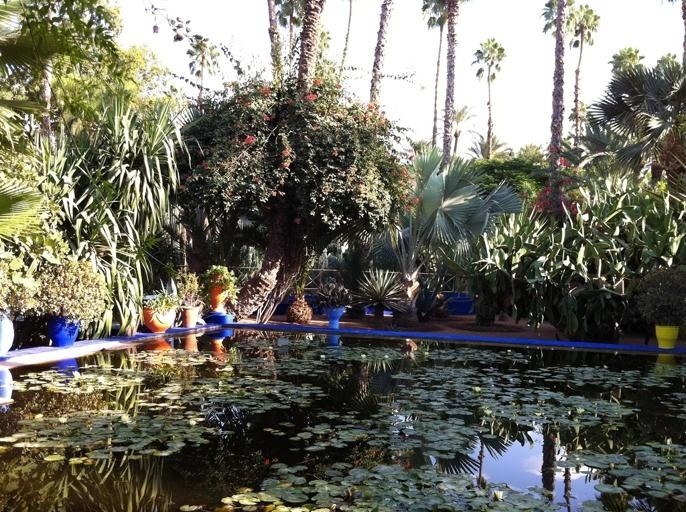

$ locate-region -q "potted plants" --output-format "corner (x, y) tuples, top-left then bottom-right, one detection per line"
(634, 264), (686, 349)
(319, 284), (352, 322)
(2, 256), (236, 356)
